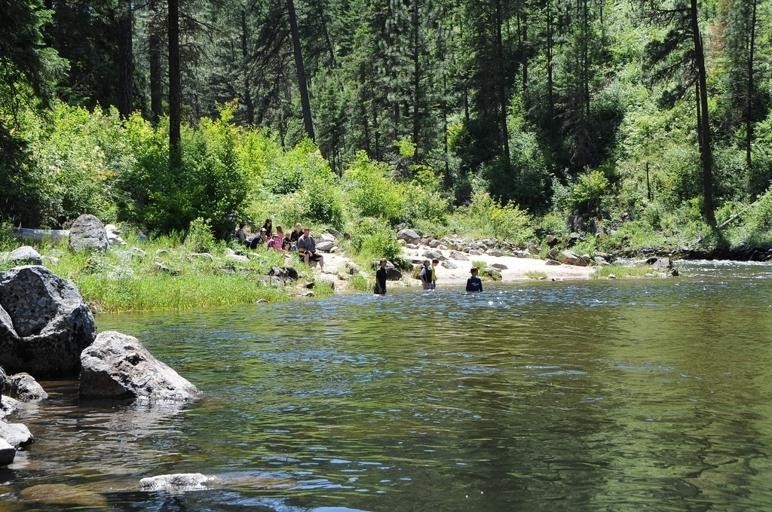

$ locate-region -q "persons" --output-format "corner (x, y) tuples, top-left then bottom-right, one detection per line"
(236, 219), (324, 273)
(375, 258), (388, 294)
(419, 260), (433, 290)
(465, 268), (484, 293)
(431, 259), (440, 290)
